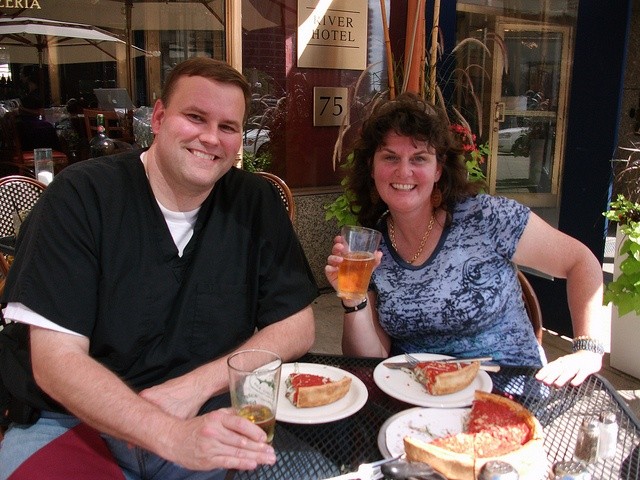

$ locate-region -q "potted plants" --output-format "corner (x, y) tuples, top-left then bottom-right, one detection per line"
(601, 193), (639, 380)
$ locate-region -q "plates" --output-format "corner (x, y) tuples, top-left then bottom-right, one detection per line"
(376, 405), (547, 480)
(243, 361), (369, 427)
(372, 352), (494, 408)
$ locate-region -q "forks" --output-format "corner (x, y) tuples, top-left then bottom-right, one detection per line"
(323, 453), (407, 480)
(404, 352), (501, 374)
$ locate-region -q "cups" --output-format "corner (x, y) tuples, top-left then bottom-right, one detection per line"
(336, 224), (383, 301)
(226, 348), (283, 447)
(33, 147), (55, 187)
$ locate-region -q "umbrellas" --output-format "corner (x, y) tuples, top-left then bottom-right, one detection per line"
(1, 2), (276, 140)
(0, 16), (156, 67)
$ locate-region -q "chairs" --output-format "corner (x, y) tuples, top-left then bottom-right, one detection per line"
(82, 108), (136, 154)
(0, 111), (68, 179)
(253, 170), (295, 225)
(516, 267), (544, 345)
(0, 174), (48, 299)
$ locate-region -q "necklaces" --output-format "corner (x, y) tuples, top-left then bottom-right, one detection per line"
(389, 216), (436, 264)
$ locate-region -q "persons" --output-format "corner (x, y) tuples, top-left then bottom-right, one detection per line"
(324, 93), (606, 410)
(0, 55), (324, 480)
(0, 81), (61, 153)
(52, 98), (87, 138)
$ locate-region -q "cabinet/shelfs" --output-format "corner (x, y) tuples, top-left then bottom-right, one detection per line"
(454, 1), (579, 209)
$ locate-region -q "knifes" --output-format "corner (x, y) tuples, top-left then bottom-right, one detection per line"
(383, 356), (493, 369)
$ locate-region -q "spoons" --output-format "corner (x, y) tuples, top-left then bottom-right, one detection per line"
(380, 459), (436, 480)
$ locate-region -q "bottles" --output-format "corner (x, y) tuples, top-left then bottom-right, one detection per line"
(525, 88), (534, 99)
(529, 87), (545, 108)
(573, 416), (600, 465)
(477, 460), (520, 480)
(598, 410), (619, 460)
(552, 460), (592, 480)
(88, 112), (116, 157)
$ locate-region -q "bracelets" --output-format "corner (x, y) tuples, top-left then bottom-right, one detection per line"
(572, 335), (604, 355)
(341, 298), (367, 313)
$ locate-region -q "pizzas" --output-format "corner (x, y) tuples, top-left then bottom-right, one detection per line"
(285, 372), (353, 409)
(404, 391), (545, 476)
(414, 360), (482, 395)
